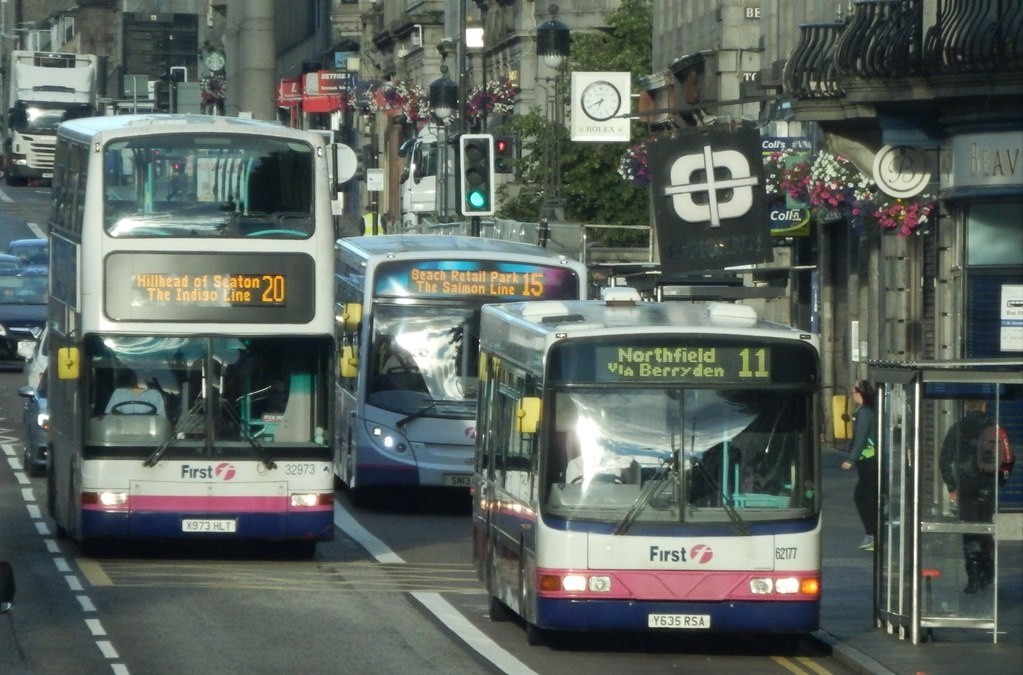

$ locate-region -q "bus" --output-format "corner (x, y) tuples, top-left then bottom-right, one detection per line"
(470, 287), (856, 648)
(45, 116), (364, 562)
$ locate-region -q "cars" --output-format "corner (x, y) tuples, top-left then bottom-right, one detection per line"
(23, 320), (52, 397)
(0, 238), (50, 366)
(17, 367), (48, 477)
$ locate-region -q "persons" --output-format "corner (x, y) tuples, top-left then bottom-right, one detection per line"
(939, 399), (1015, 593)
(841, 381), (889, 549)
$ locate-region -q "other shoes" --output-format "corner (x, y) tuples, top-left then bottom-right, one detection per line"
(964, 579), (982, 593)
(859, 534), (874, 550)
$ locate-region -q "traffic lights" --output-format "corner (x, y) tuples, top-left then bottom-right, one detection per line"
(158, 55), (172, 82)
(155, 82), (170, 115)
(493, 138), (515, 174)
(173, 160), (183, 172)
(356, 144), (374, 183)
(459, 134), (494, 217)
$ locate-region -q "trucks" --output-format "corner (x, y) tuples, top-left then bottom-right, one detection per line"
(3, 51), (100, 185)
(396, 122), (454, 234)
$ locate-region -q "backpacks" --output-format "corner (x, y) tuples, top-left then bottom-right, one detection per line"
(976, 417), (1013, 482)
